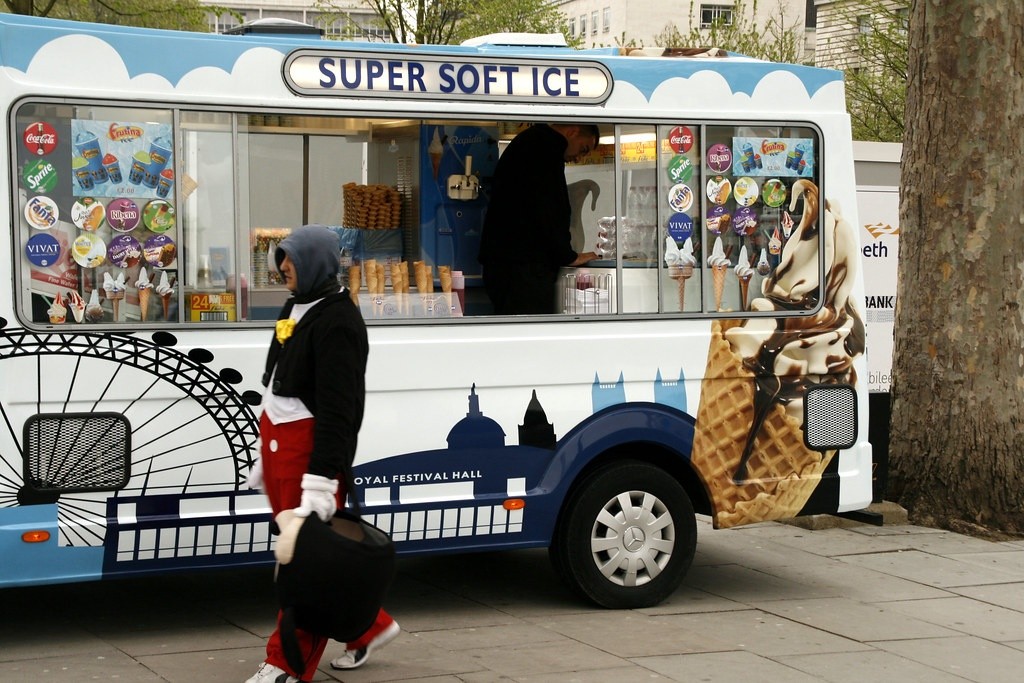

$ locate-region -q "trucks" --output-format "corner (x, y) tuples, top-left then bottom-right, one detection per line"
(1, 15), (874, 611)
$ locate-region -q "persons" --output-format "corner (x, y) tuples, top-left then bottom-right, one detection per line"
(481, 125), (599, 313)
(246, 223), (401, 683)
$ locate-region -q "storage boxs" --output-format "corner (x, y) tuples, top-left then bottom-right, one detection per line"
(340, 228), (404, 288)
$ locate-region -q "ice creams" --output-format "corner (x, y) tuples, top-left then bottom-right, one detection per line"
(156, 271), (175, 320)
(664, 238), (754, 312)
(48, 292), (67, 324)
(103, 272), (125, 322)
(135, 267), (154, 320)
(84, 289), (103, 324)
(67, 288), (86, 323)
(691, 180), (865, 530)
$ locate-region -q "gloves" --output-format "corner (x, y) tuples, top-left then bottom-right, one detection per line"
(248, 437), (263, 488)
(293, 473), (339, 522)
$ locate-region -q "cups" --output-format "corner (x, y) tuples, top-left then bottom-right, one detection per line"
(793, 149), (805, 170)
(142, 142), (173, 188)
(128, 156), (151, 185)
(785, 155), (795, 169)
(156, 173), (173, 198)
(101, 159), (122, 184)
(796, 163), (806, 175)
(742, 146), (756, 170)
(74, 137), (109, 184)
(753, 157), (762, 169)
(73, 162), (94, 191)
(740, 158), (750, 173)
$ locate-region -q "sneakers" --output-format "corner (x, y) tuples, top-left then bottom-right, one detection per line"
(330, 620), (400, 667)
(244, 662), (303, 683)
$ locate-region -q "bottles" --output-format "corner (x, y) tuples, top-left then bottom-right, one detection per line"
(35, 123), (45, 156)
(678, 127), (684, 153)
